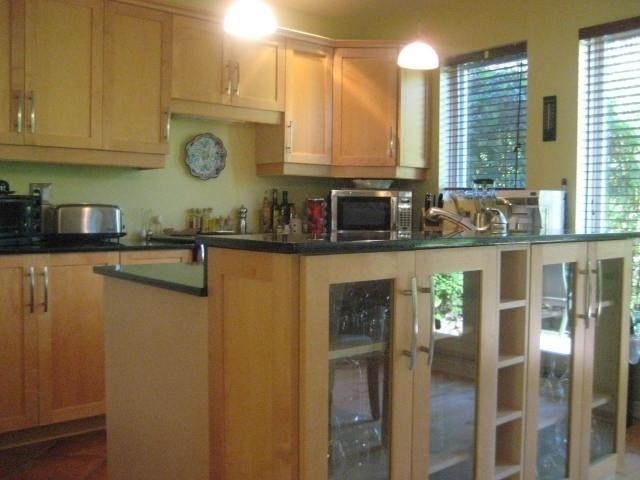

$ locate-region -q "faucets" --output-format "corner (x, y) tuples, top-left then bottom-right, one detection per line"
(476, 196), (512, 227)
(425, 205), (490, 232)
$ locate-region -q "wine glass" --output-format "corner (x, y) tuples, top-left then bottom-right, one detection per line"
(559, 360), (569, 401)
(540, 354), (564, 409)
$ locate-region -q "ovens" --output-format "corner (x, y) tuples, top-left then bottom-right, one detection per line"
(0, 194), (43, 253)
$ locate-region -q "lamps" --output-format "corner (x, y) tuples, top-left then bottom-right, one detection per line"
(222, 2), (276, 42)
(396, 21), (439, 70)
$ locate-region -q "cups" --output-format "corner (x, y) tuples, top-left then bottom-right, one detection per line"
(330, 298), (390, 343)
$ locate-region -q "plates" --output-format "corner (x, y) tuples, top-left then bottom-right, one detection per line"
(351, 179), (397, 189)
(216, 230), (235, 234)
(184, 132), (228, 181)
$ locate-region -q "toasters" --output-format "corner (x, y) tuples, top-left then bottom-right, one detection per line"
(49, 203), (128, 244)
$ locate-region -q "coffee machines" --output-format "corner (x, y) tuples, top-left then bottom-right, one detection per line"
(494, 189), (565, 236)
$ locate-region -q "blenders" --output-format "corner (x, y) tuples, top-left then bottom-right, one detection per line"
(473, 177), (495, 239)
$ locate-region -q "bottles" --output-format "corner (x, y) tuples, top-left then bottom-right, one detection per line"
(262, 189), (271, 233)
(208, 207), (216, 231)
(201, 208), (208, 233)
(226, 216), (234, 231)
(239, 205), (247, 234)
(279, 191), (289, 217)
(196, 208), (201, 231)
(217, 216), (225, 230)
(306, 198), (324, 238)
(461, 210), (474, 239)
(191, 208), (196, 229)
(272, 189), (280, 232)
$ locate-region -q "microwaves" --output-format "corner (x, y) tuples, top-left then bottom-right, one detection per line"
(329, 190), (412, 240)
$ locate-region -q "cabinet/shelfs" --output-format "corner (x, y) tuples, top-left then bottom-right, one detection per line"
(253, 27), (333, 177)
(332, 37), (430, 182)
(195, 233), (524, 480)
(0, 247), (122, 451)
(170, 3), (287, 127)
(1, 0), (102, 166)
(121, 245), (193, 266)
(101, 3), (172, 169)
(525, 232), (640, 479)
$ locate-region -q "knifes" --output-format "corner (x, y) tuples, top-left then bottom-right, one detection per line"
(425, 192), (444, 227)
